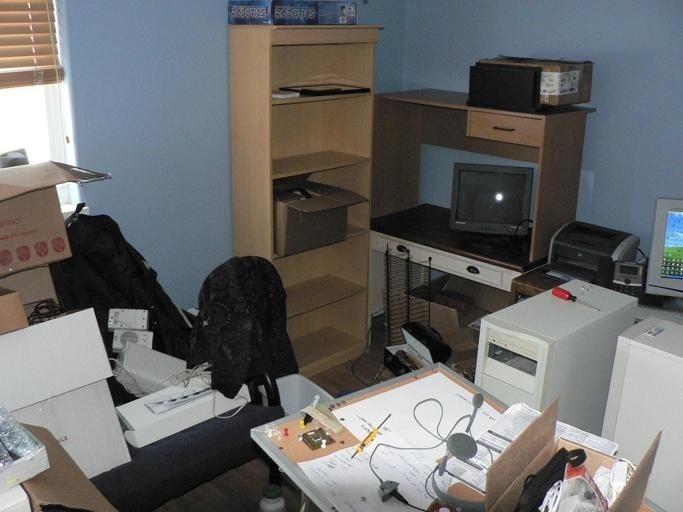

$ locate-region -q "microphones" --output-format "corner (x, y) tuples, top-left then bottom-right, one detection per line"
(463, 393), (484, 435)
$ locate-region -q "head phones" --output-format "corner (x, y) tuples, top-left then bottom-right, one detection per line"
(430, 433), (498, 512)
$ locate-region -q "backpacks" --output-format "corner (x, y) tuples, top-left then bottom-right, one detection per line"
(47, 201), (194, 384)
(201, 255), (300, 401)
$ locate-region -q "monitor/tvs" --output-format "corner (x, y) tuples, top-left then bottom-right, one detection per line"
(450, 161), (534, 248)
(645, 197), (683, 310)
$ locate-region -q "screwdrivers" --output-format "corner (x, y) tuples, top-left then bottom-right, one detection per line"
(350, 428), (380, 459)
(552, 286), (600, 312)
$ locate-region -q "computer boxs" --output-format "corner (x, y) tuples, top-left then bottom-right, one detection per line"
(473, 278), (638, 438)
(599, 315), (683, 512)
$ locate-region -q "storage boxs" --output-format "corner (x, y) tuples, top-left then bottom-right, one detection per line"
(1, 310), (130, 479)
(0, 159), (107, 274)
(382, 277), (508, 351)
(484, 394), (664, 512)
(0, 263), (65, 325)
(0, 288), (29, 333)
(13, 421), (119, 512)
(477, 55), (593, 105)
(273, 181), (368, 256)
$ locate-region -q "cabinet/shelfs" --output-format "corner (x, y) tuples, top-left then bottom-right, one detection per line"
(372, 88), (597, 268)
(225, 21), (381, 379)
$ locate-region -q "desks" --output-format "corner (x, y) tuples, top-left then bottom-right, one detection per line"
(509, 265), (669, 319)
(249, 360), (511, 511)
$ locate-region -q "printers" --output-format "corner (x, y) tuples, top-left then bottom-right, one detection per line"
(547, 220), (639, 289)
